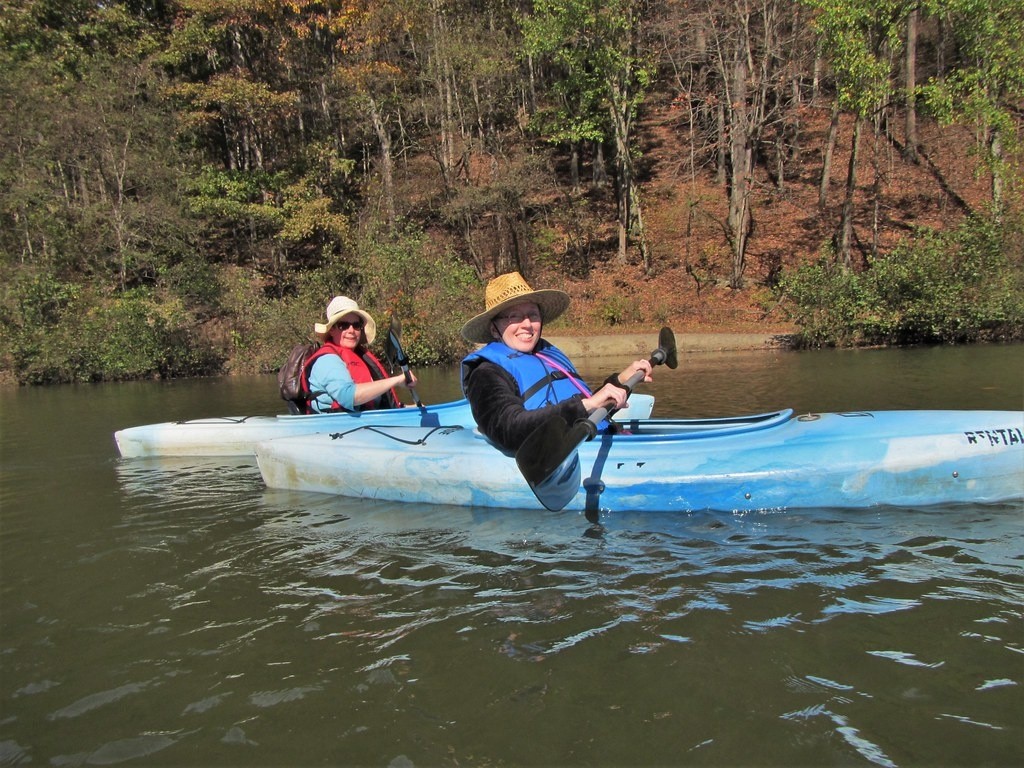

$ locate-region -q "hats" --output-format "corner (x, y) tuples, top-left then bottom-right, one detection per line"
(461, 271), (571, 344)
(314, 296), (376, 346)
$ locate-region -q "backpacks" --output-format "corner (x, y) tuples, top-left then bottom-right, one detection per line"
(276, 340), (320, 410)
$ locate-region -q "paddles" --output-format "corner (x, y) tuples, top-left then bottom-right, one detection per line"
(379, 313), (425, 410)
(514, 326), (679, 513)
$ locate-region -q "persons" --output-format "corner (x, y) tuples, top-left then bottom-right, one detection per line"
(302, 296), (424, 415)
(459, 272), (652, 450)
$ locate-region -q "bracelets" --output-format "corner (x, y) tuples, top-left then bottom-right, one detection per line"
(399, 402), (405, 408)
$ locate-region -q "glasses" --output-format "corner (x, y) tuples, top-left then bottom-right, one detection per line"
(332, 321), (365, 331)
(493, 310), (542, 323)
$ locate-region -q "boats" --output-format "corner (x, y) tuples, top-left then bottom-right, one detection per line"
(252, 402), (1024, 518)
(112, 390), (656, 459)
